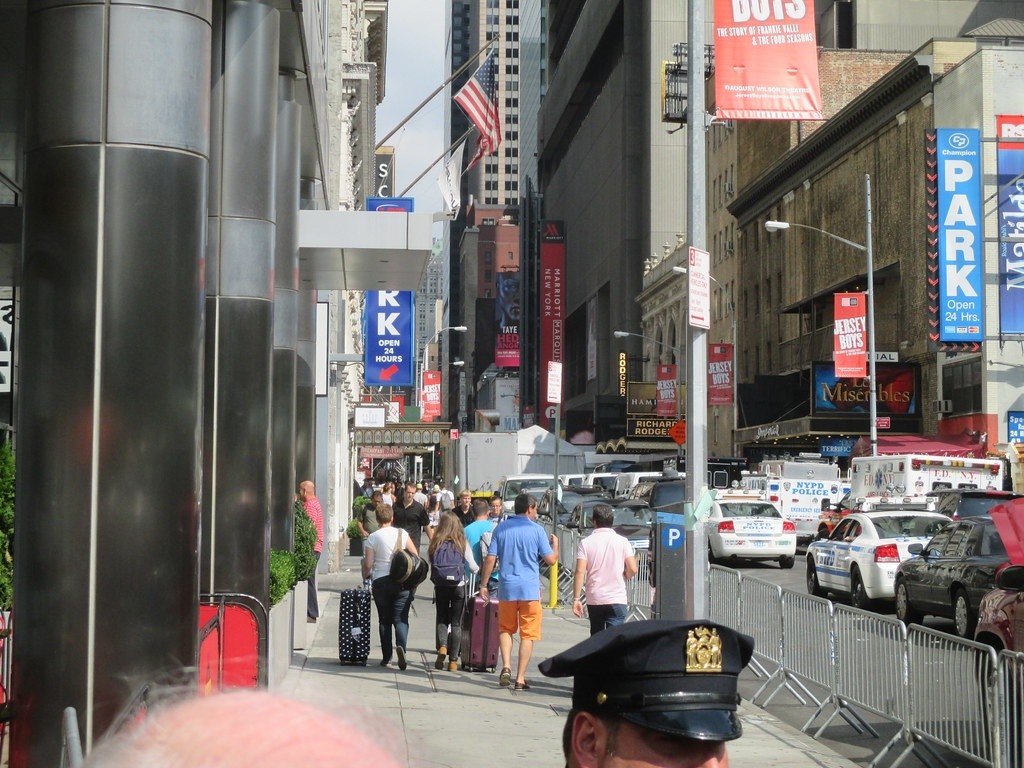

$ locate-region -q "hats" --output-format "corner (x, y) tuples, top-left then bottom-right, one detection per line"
(416, 484), (423, 489)
(433, 485), (439, 492)
(537, 619), (754, 739)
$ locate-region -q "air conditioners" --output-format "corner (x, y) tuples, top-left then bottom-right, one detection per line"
(933, 399), (953, 414)
(723, 183), (731, 194)
(724, 240), (732, 251)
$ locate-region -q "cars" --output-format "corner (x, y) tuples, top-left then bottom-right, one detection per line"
(537, 481), (796, 580)
(806, 512), (1024, 760)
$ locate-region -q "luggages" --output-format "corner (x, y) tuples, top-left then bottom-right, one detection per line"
(460, 573), (499, 674)
(338, 578), (370, 666)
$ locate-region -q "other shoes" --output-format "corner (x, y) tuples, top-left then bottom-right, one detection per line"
(380, 656), (390, 666)
(498, 667), (510, 685)
(396, 646), (407, 671)
(448, 659), (458, 672)
(435, 646), (446, 670)
(515, 681), (531, 691)
(306, 614), (317, 623)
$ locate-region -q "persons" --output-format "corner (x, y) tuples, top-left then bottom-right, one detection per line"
(572, 504), (638, 637)
(539, 619), (754, 768)
(356, 479), (512, 672)
(294, 481), (323, 622)
(479, 494), (559, 690)
(495, 272), (520, 332)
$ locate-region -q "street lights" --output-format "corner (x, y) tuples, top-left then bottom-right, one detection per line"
(614, 330), (681, 458)
(417, 326), (466, 420)
(672, 265), (738, 457)
(766, 172), (878, 457)
(501, 394), (537, 424)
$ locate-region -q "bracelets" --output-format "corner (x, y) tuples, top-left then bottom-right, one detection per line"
(479, 585), (487, 588)
(574, 598), (580, 601)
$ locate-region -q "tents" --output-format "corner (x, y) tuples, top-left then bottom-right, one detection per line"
(851, 434), (983, 459)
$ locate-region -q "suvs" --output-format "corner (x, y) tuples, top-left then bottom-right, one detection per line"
(924, 490), (1024, 520)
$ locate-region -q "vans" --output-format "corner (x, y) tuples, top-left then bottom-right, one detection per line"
(497, 472), (688, 520)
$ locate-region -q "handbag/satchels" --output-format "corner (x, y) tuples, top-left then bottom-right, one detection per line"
(389, 528), (428, 587)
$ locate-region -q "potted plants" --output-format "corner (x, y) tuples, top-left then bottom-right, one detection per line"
(270, 495), (316, 684)
(346, 495), (372, 556)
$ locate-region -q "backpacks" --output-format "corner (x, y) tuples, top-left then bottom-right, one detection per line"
(441, 490), (451, 510)
(428, 494), (437, 512)
(430, 539), (463, 586)
(481, 521), (501, 574)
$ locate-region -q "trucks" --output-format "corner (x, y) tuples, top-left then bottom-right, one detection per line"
(678, 457), (845, 544)
(815, 431), (1004, 540)
(438, 432), (518, 509)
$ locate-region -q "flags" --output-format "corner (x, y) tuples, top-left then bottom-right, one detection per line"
(433, 141), (466, 222)
(453, 50), (502, 159)
